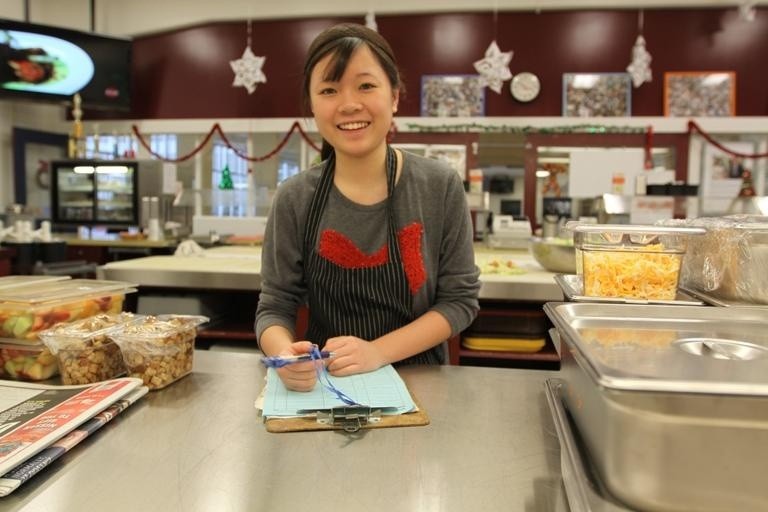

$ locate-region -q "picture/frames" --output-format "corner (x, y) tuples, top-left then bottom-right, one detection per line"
(562, 72), (632, 117)
(662, 70), (736, 117)
(420, 72), (485, 117)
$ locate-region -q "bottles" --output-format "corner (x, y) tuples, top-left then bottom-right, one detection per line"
(739, 170), (755, 196)
(219, 165), (234, 190)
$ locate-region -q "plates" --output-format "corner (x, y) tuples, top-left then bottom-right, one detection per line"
(463, 335), (545, 353)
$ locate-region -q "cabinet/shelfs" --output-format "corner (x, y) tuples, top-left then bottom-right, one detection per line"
(95, 270), (563, 372)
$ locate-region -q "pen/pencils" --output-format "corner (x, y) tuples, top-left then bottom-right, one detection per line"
(261, 351), (337, 369)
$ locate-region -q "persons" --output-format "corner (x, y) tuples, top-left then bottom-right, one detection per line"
(252, 19), (482, 394)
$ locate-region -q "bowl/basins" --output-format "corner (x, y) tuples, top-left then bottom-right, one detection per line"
(524, 235), (576, 275)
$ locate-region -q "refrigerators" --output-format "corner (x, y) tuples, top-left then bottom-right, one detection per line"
(49, 159), (162, 228)
